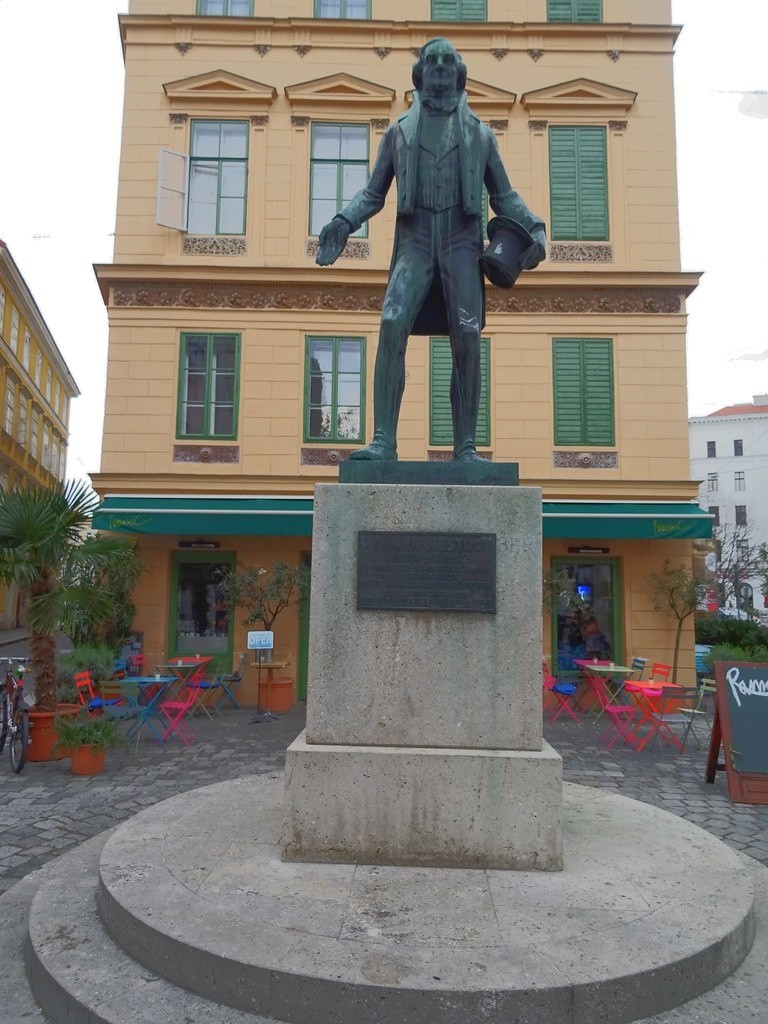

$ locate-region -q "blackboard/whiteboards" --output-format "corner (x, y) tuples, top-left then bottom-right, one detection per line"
(713, 660), (768, 774)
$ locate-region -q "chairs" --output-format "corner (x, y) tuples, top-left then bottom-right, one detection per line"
(678, 676), (720, 755)
(183, 656), (224, 721)
(588, 676), (641, 752)
(634, 663), (674, 747)
(216, 652), (251, 716)
(541, 662), (581, 724)
(131, 655), (160, 708)
(75, 671), (125, 721)
(153, 667), (205, 750)
(98, 679), (149, 757)
(609, 657), (647, 727)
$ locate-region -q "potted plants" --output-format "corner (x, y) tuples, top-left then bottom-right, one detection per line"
(211, 554), (312, 716)
(0, 477), (139, 763)
(641, 555), (718, 715)
(49, 700), (135, 776)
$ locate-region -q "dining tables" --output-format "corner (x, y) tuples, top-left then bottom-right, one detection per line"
(623, 679), (688, 756)
(116, 675), (179, 750)
(572, 659), (617, 719)
(582, 663), (637, 727)
(166, 656), (216, 718)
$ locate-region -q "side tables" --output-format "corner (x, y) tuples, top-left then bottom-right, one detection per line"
(248, 660), (292, 721)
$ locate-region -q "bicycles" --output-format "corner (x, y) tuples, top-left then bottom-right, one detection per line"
(0, 656), (42, 774)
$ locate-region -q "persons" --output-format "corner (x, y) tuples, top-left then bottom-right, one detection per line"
(313, 36), (549, 465)
(188, 565), (209, 637)
(209, 569), (228, 637)
